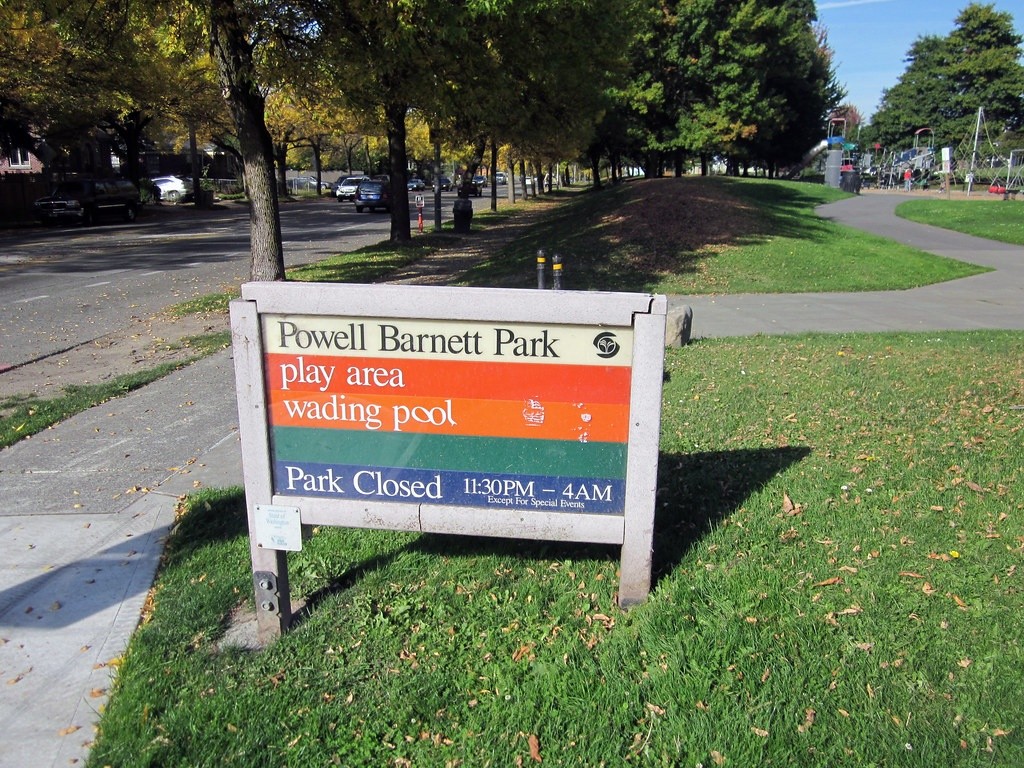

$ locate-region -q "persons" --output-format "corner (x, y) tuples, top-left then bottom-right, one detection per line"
(904, 166), (913, 192)
(544, 173), (549, 188)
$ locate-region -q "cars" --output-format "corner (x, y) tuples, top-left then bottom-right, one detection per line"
(458, 176), (487, 196)
(431, 178), (452, 192)
(331, 175), (390, 213)
(407, 179), (425, 191)
(525, 173), (573, 188)
(289, 175), (331, 192)
(151, 175), (194, 200)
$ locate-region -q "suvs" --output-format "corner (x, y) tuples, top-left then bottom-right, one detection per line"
(34, 176), (140, 227)
(496, 172), (506, 185)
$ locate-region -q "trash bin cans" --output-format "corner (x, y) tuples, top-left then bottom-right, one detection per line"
(201, 190), (213, 208)
(452, 200), (474, 232)
(840, 171), (862, 194)
